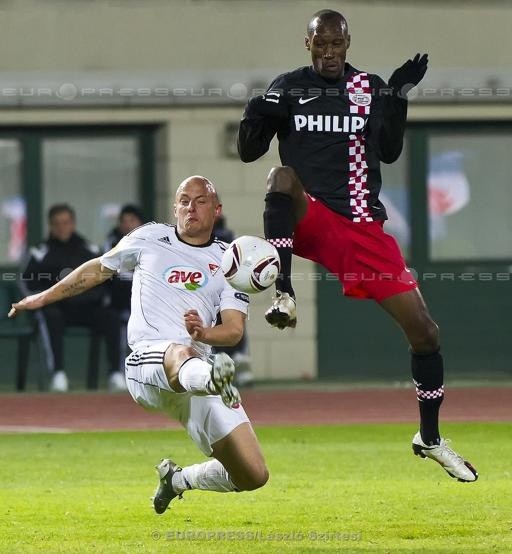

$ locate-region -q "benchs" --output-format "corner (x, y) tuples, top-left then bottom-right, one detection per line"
(0, 321), (119, 394)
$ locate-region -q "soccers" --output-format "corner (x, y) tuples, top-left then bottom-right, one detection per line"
(221, 235), (281, 293)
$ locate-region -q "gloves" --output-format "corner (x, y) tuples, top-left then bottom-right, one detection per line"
(250, 90), (289, 120)
(389, 53), (428, 97)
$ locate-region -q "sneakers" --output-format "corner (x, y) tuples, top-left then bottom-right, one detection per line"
(153, 458), (182, 514)
(411, 429), (478, 482)
(211, 352), (242, 409)
(265, 290), (298, 330)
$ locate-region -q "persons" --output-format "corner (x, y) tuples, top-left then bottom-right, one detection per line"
(15, 203), (127, 395)
(237, 9), (478, 482)
(100, 203), (156, 355)
(6, 175), (269, 514)
(212, 216), (254, 388)
